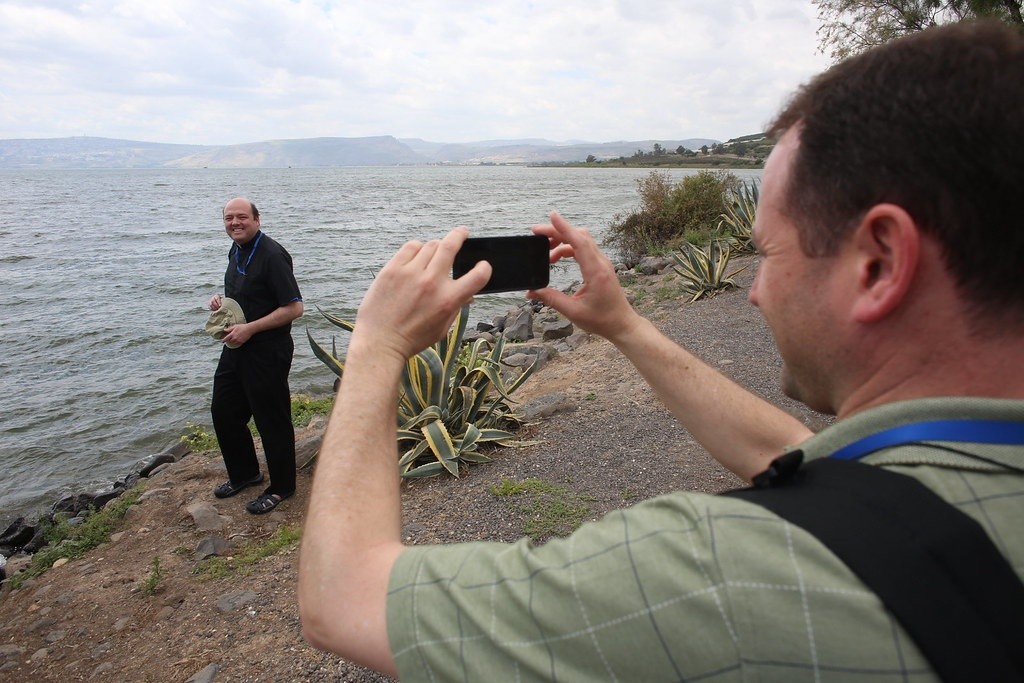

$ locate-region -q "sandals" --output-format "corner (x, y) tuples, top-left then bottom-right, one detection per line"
(245, 485), (296, 514)
(214, 471), (263, 498)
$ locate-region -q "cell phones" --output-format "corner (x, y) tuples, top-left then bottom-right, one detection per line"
(452, 235), (550, 294)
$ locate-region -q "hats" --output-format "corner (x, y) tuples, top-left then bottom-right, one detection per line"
(205, 297), (246, 349)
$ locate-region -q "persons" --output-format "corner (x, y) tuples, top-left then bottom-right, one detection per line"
(294, 19), (1023, 681)
(207, 197), (305, 513)
